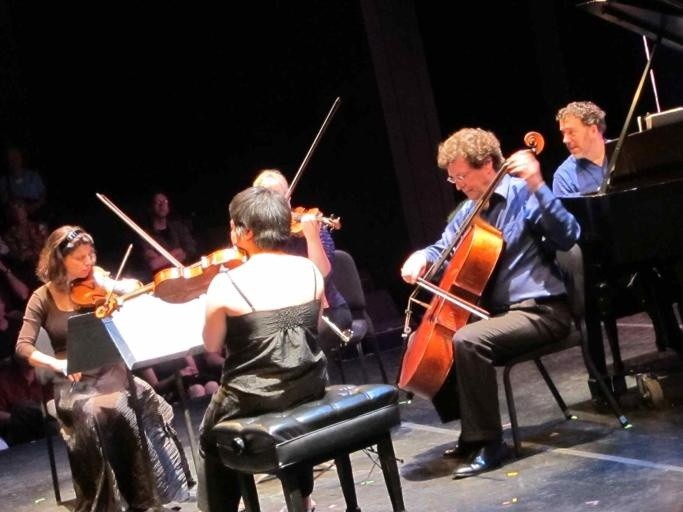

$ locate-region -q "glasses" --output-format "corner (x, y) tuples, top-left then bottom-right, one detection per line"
(446, 173), (470, 184)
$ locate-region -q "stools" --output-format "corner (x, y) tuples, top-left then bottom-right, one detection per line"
(205, 381), (405, 512)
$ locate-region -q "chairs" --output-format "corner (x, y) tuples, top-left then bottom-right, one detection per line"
(326, 250), (390, 385)
(492, 242), (643, 458)
(19, 325), (171, 507)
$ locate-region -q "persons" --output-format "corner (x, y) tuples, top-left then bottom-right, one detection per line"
(202, 171), (351, 511)
(1, 191), (198, 512)
(552, 101), (612, 199)
(400, 127), (582, 477)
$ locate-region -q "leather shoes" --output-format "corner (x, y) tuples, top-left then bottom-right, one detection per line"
(443, 439), (511, 477)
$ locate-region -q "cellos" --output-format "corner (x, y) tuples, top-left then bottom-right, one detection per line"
(394, 132), (546, 399)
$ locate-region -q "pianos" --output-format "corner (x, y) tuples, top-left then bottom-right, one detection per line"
(556, 0), (683, 412)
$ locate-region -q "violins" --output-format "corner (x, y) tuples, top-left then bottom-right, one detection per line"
(94, 247), (248, 321)
(288, 203), (342, 238)
(69, 263), (145, 310)
